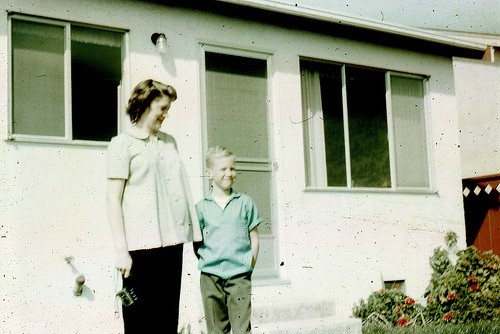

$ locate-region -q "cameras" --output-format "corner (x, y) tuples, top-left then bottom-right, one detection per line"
(116, 287), (138, 307)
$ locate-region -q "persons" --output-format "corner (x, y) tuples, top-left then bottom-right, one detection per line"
(193, 145), (264, 334)
(104, 79), (203, 334)
